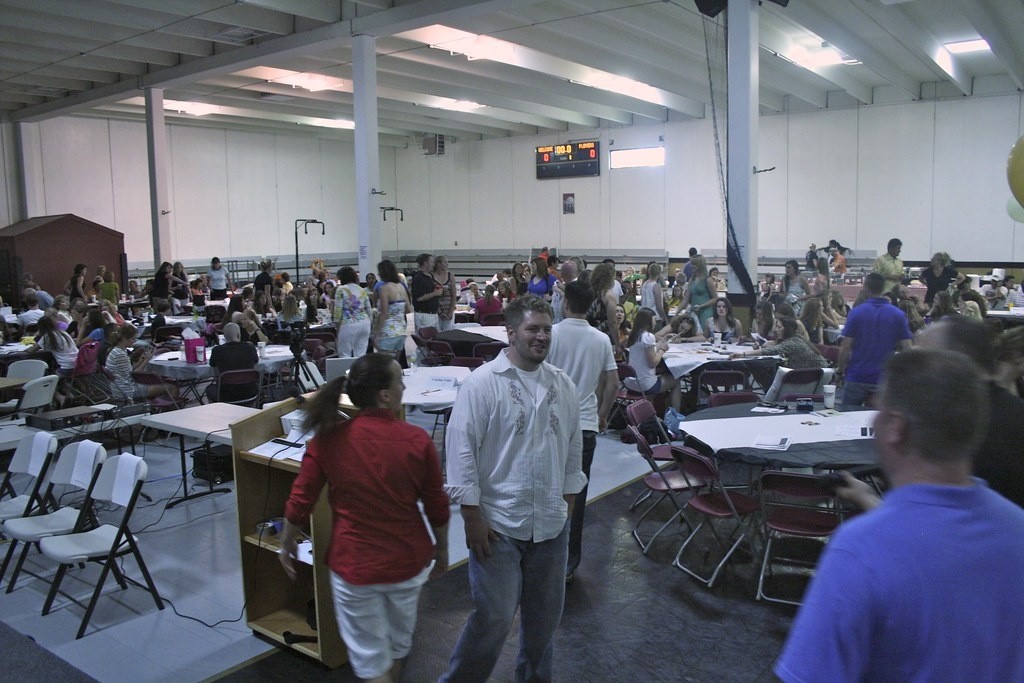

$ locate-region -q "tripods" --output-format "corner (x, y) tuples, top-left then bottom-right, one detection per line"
(282, 343), (321, 400)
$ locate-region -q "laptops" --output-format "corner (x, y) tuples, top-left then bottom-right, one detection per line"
(326, 357), (358, 393)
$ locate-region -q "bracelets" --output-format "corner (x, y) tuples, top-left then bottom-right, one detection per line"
(744, 352), (745, 357)
(335, 327), (338, 330)
(438, 312), (443, 315)
(659, 348), (665, 352)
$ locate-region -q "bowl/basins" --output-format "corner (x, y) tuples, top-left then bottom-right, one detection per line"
(1009, 307), (1024, 316)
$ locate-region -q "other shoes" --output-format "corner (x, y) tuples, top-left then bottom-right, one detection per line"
(565, 568), (574, 583)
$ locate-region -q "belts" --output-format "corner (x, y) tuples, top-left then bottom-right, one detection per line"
(414, 308), (436, 314)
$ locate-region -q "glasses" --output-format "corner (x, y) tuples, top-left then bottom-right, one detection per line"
(683, 318), (694, 321)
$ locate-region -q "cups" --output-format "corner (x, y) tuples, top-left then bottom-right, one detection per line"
(142, 312), (148, 322)
(326, 314), (332, 323)
(1009, 302), (1013, 311)
(207, 289), (210, 295)
(714, 334), (722, 348)
(122, 294), (126, 303)
(822, 385), (836, 409)
(196, 346), (204, 365)
(91, 295), (96, 303)
(502, 297), (508, 308)
(178, 284), (182, 287)
(265, 313), (272, 318)
(129, 295), (134, 303)
(258, 342), (266, 359)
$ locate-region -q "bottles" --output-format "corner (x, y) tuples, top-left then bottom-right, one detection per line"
(180, 341), (186, 361)
(410, 351), (417, 373)
(483, 361), (486, 365)
(128, 308), (132, 319)
(193, 306), (198, 320)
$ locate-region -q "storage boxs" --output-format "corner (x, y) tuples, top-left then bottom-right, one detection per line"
(280, 408), (319, 436)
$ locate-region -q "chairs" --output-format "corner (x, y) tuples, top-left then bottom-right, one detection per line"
(0, 305), (851, 639)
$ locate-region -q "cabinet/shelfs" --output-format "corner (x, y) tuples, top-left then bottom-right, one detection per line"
(227, 390), (407, 668)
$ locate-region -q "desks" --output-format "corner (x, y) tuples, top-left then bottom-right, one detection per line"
(0, 273), (1024, 574)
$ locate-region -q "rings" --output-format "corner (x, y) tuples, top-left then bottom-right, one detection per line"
(488, 529), (494, 536)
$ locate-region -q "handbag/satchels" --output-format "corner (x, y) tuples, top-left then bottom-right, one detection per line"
(705, 276), (718, 319)
(637, 418), (673, 445)
(611, 401), (635, 429)
(663, 407), (687, 440)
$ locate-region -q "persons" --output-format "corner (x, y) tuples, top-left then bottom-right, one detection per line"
(280, 354), (452, 683)
(374, 260), (414, 363)
(728, 317), (830, 369)
(545, 277), (619, 580)
(206, 322), (260, 408)
(105, 323), (179, 412)
(412, 254), (448, 337)
(444, 296), (588, 683)
(333, 266), (373, 359)
(771, 349), (1024, 683)
(0, 238), (1023, 506)
(622, 308), (682, 414)
(427, 256), (457, 330)
